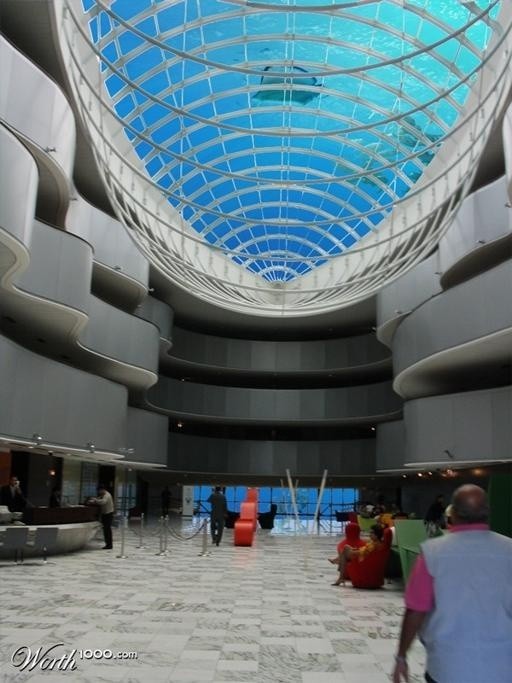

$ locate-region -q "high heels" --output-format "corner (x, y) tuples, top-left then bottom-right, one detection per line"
(328, 558), (340, 565)
(332, 578), (346, 587)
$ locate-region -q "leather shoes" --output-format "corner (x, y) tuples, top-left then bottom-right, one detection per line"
(102, 544), (113, 549)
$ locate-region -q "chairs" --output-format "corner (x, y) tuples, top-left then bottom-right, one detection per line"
(337, 524), (367, 555)
(257, 504), (276, 529)
(0, 528), (58, 563)
(345, 529), (392, 589)
(225, 512), (240, 528)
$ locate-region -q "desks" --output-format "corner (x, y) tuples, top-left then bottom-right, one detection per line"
(394, 518), (429, 587)
(356, 514), (400, 578)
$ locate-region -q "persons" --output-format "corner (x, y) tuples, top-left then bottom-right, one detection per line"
(328, 483), (512, 683)
(89, 484), (114, 550)
(208, 485), (228, 547)
(161, 486), (172, 514)
(49, 487), (61, 507)
(0, 474), (27, 512)
